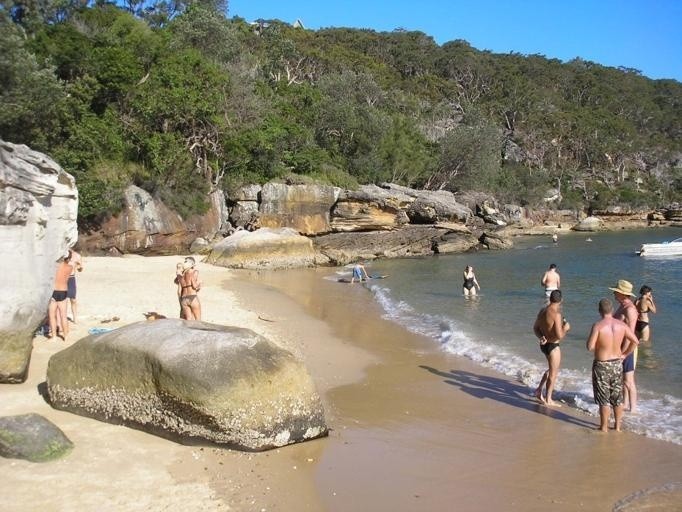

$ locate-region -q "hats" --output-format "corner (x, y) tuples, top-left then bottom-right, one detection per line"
(608, 280), (637, 297)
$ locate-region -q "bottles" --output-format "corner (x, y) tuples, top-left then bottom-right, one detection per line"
(563, 316), (569, 332)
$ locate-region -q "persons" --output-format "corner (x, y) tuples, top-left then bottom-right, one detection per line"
(49, 250), (74, 344)
(586, 298), (640, 432)
(67, 250), (84, 323)
(540, 263), (561, 298)
(633, 284), (656, 343)
(174, 263), (185, 319)
(178, 257), (204, 321)
(608, 279), (639, 414)
(551, 233), (559, 244)
(463, 264), (480, 299)
(532, 290), (570, 408)
(350, 262), (369, 285)
